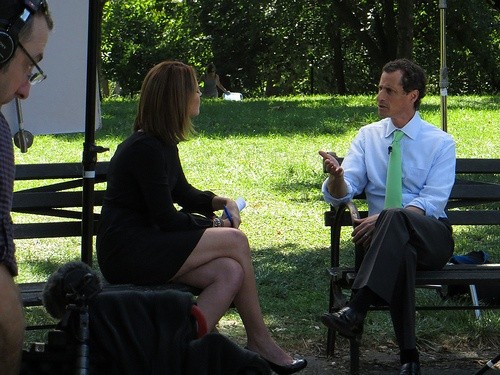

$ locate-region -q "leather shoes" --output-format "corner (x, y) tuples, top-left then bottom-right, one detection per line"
(319, 305), (366, 340)
(396, 361), (422, 375)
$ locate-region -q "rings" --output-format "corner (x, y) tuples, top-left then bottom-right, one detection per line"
(365, 234), (368, 236)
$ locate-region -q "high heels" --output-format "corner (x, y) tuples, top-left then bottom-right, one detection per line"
(244, 345), (307, 375)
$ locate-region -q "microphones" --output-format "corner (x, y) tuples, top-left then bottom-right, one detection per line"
(43, 260), (101, 318)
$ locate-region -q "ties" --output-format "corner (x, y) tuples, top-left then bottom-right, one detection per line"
(384, 130), (405, 209)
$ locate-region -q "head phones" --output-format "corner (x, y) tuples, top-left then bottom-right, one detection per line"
(0, 0), (43, 68)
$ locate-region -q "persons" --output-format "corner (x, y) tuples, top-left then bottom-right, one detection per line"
(0, 0), (53, 375)
(96, 62), (309, 375)
(197, 63), (230, 99)
(319, 59), (456, 375)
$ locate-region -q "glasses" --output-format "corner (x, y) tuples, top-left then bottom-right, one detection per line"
(193, 88), (201, 95)
(17, 40), (47, 85)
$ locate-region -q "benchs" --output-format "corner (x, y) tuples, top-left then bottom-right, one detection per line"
(10, 162), (217, 329)
(323, 151), (500, 375)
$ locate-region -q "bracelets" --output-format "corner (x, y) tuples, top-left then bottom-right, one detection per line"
(220, 219), (224, 227)
(214, 218), (221, 227)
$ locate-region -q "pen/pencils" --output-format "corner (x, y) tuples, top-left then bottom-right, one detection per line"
(223, 204), (233, 223)
